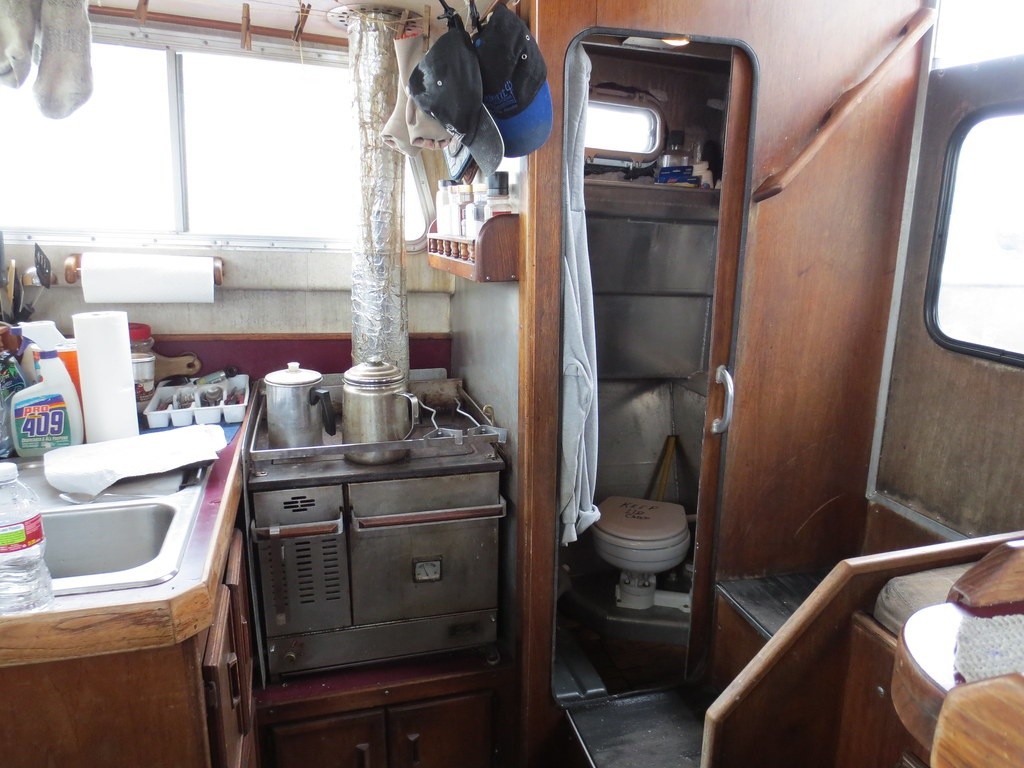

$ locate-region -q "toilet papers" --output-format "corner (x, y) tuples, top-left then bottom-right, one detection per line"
(72, 309), (141, 442)
(80, 251), (216, 304)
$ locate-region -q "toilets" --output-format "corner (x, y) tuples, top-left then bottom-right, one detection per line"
(591, 377), (696, 608)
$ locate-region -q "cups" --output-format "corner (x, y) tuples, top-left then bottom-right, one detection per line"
(31, 338), (86, 444)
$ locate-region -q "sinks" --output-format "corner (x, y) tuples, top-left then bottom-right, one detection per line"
(40, 504), (187, 591)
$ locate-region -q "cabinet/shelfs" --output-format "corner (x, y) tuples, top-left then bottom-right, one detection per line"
(254, 659), (498, 767)
(0, 521), (256, 768)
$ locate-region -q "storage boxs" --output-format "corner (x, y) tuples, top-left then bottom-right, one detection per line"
(142, 373), (249, 429)
(658, 166), (698, 187)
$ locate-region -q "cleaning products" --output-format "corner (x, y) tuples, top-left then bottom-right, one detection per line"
(13, 337), (81, 462)
(0, 344), (29, 457)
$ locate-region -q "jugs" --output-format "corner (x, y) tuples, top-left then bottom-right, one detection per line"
(342, 356), (420, 465)
(265, 362), (336, 458)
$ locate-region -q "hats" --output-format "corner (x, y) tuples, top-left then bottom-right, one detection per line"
(470, 6), (554, 158)
(405, 10), (505, 180)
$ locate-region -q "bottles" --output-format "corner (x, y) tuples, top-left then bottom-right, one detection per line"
(690, 161), (713, 188)
(436, 169), (511, 239)
(126, 322), (156, 414)
(0, 461), (55, 612)
(658, 129), (689, 167)
